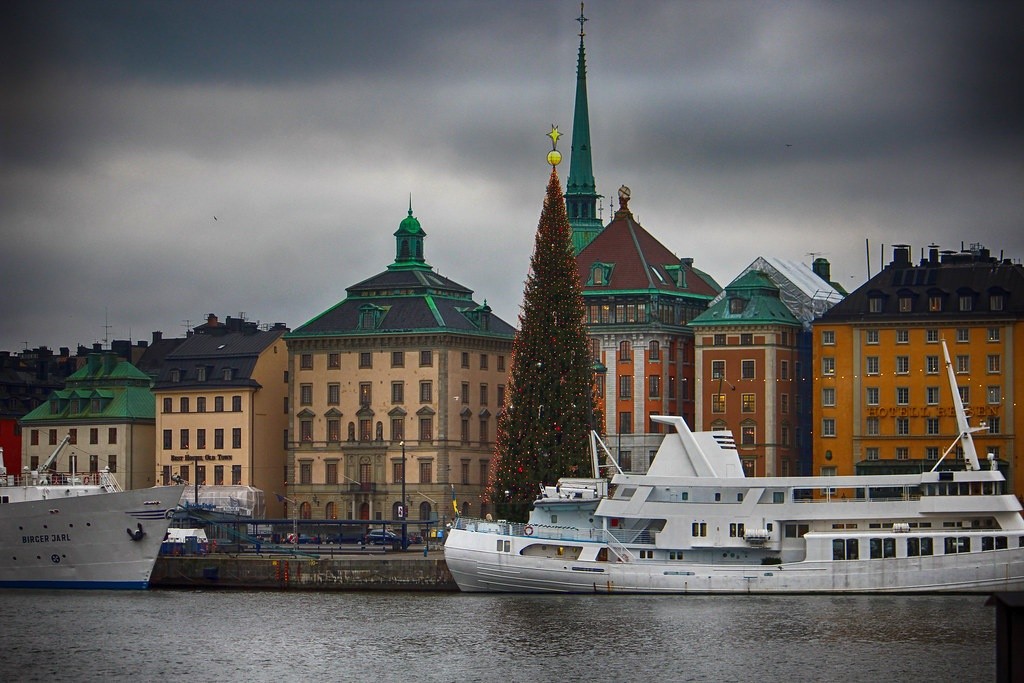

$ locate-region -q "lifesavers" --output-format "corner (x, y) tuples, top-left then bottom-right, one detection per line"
(524, 526), (534, 535)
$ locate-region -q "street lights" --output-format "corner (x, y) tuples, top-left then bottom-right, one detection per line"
(399, 440), (408, 552)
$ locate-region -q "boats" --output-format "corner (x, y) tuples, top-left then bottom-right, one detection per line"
(0, 451), (186, 591)
(445, 336), (1024, 592)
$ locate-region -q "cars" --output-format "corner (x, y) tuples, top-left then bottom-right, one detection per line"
(365, 529), (401, 547)
